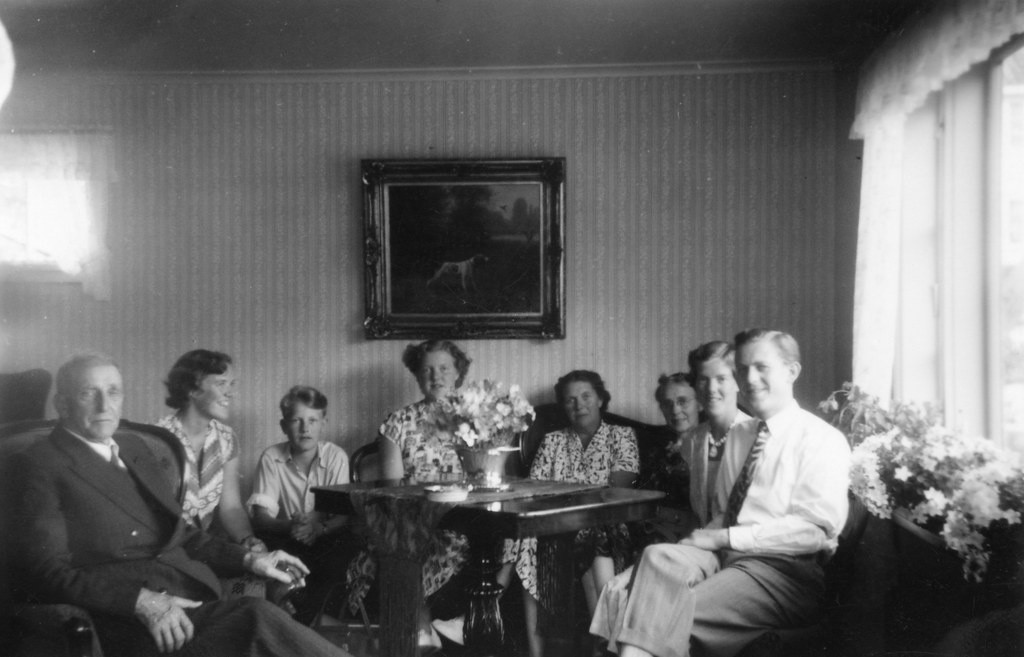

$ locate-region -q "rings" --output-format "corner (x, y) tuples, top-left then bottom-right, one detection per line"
(284, 568), (289, 572)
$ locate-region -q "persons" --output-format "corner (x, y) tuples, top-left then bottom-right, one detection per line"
(589, 341), (751, 657)
(616, 329), (851, 657)
(376, 340), (471, 648)
(22, 350), (357, 657)
(159, 349), (334, 630)
(432, 370), (639, 657)
(244, 385), (356, 544)
(574, 371), (703, 617)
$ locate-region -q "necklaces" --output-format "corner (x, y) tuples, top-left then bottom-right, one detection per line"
(194, 441), (203, 452)
(708, 419), (733, 457)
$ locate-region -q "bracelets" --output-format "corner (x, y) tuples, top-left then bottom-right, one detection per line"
(322, 522), (330, 534)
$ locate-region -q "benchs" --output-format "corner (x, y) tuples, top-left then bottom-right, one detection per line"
(516, 401), (752, 481)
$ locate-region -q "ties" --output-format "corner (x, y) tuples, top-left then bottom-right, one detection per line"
(718, 421), (768, 557)
(109, 445), (121, 467)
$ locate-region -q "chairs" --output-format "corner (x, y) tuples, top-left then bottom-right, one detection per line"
(739, 431), (888, 657)
(0, 416), (191, 657)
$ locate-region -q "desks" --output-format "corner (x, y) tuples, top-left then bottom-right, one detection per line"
(309, 476), (667, 657)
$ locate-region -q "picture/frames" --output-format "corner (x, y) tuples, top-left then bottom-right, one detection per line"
(358, 157), (568, 342)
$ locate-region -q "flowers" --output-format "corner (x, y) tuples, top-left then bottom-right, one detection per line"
(815, 379), (1024, 582)
(434, 382), (537, 454)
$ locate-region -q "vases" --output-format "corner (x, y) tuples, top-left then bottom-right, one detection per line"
(889, 501), (1011, 601)
(458, 443), (514, 492)
